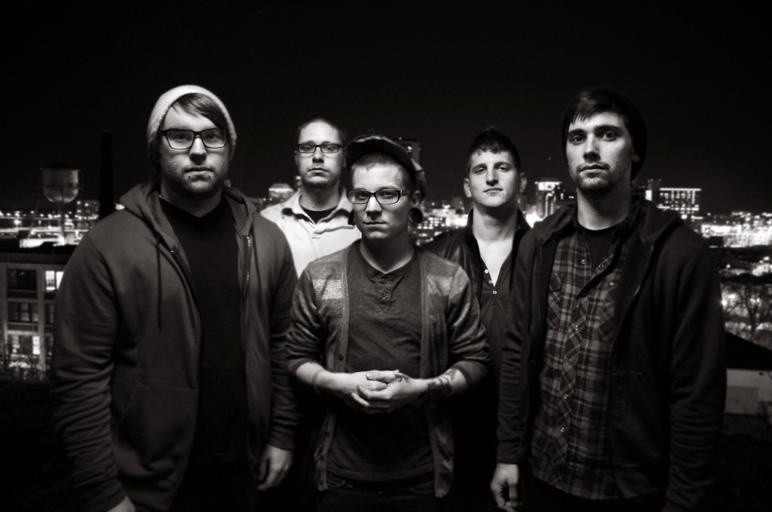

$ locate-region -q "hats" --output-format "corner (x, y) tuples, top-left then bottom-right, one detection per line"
(345, 134), (418, 189)
(145, 83), (238, 163)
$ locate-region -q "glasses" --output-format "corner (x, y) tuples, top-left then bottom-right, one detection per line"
(345, 187), (412, 206)
(297, 142), (342, 153)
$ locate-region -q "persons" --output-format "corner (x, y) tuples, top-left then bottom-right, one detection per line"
(490, 88), (728, 511)
(282, 134), (489, 512)
(47, 84), (299, 512)
(422, 129), (530, 329)
(259, 114), (363, 279)
